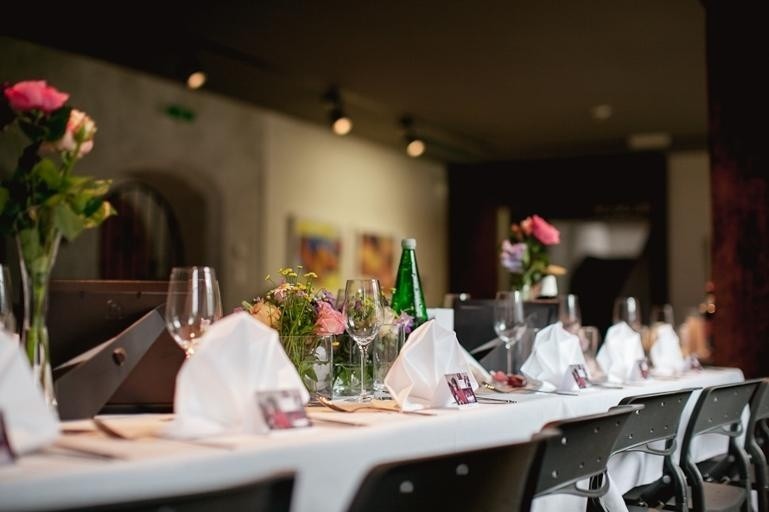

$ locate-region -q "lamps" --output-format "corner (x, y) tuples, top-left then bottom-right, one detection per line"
(400, 112), (425, 160)
(318, 84), (354, 138)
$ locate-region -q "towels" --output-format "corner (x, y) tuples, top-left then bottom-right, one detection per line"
(596, 321), (647, 385)
(0, 329), (63, 461)
(384, 319), (492, 413)
(520, 321), (595, 392)
(165, 311), (310, 440)
(649, 326), (688, 377)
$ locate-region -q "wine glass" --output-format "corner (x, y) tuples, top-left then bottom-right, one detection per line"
(0, 263), (13, 328)
(649, 305), (675, 330)
(559, 295), (582, 334)
(164, 266), (222, 359)
(341, 280), (383, 403)
(613, 296), (642, 333)
(493, 290), (527, 376)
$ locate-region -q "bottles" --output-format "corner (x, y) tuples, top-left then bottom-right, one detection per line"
(388, 237), (429, 321)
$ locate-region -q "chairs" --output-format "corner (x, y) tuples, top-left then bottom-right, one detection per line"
(345, 437), (549, 511)
(746, 377), (767, 512)
(619, 385), (703, 512)
(55, 468), (296, 511)
(535, 404), (645, 511)
(680, 379), (763, 512)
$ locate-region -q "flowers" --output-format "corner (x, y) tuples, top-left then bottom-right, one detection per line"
(321, 286), (415, 396)
(1, 77), (119, 363)
(233, 262), (349, 387)
(499, 211), (568, 294)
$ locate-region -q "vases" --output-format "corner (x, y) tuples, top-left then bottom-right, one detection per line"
(334, 335), (375, 401)
(10, 227), (60, 425)
(280, 334), (317, 396)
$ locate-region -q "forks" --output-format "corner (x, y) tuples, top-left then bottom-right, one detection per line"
(319, 397), (440, 417)
(93, 416), (236, 450)
